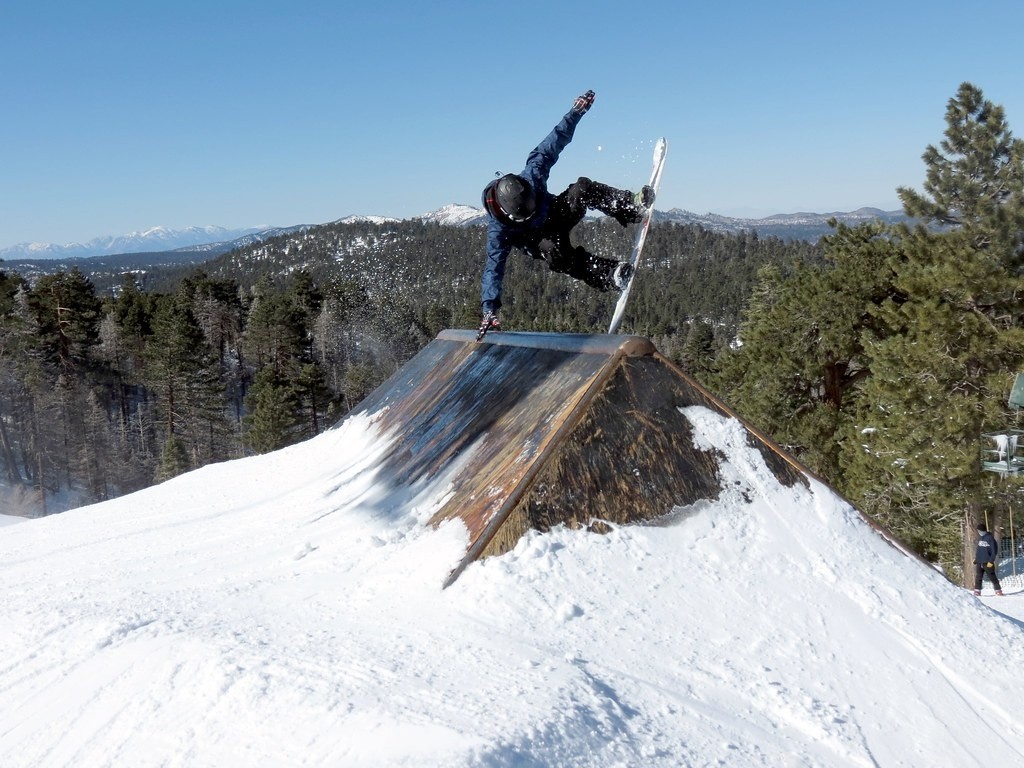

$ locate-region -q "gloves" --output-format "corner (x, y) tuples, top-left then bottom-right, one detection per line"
(986, 562), (994, 568)
(475, 301), (501, 342)
(572, 89), (596, 117)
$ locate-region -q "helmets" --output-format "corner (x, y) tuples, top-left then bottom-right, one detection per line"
(497, 173), (539, 219)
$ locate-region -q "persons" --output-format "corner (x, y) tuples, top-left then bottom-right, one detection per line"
(972, 524), (1003, 596)
(474, 89), (656, 340)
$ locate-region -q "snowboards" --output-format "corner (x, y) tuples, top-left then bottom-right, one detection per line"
(608, 135), (669, 335)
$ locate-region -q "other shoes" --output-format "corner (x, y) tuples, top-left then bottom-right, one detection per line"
(996, 590), (1002, 596)
(609, 264), (632, 291)
(973, 590), (980, 596)
(629, 187), (654, 225)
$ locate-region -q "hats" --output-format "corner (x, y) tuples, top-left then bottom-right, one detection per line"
(977, 524), (986, 531)
